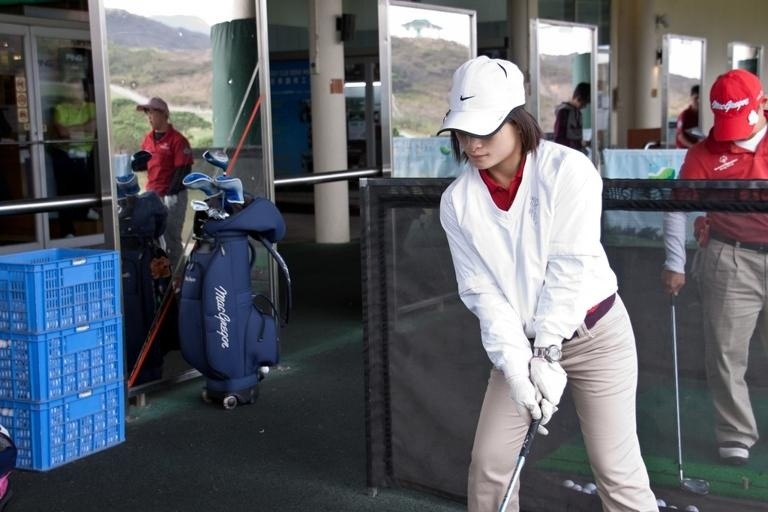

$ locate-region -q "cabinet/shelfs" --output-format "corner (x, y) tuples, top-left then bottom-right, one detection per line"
(0, 14), (105, 254)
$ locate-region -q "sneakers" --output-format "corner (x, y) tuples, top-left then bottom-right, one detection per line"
(716, 440), (750, 464)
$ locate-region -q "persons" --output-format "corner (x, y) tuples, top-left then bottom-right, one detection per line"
(132, 94), (193, 294)
(663, 68), (767, 461)
(674, 82), (699, 148)
(436, 53), (660, 512)
(549, 82), (592, 155)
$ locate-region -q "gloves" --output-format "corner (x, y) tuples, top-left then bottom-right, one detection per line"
(163, 195), (179, 208)
(505, 373), (558, 436)
(132, 151), (152, 163)
(530, 356), (567, 406)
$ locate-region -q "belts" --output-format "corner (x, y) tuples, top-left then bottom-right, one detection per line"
(562, 292), (616, 345)
(705, 231), (768, 253)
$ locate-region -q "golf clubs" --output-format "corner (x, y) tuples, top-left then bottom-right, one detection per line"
(182, 150), (244, 221)
(671, 292), (709, 494)
(112, 154), (139, 214)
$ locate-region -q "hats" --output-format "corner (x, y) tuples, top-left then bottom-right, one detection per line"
(136, 96), (168, 115)
(710, 68), (765, 143)
(436, 54), (526, 139)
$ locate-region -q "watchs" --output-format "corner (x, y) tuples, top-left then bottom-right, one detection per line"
(534, 346), (564, 362)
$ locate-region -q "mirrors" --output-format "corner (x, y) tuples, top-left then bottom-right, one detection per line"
(87, 0), (282, 399)
(726, 41), (765, 82)
(377, 0), (479, 317)
(660, 34), (707, 149)
(529, 18), (601, 168)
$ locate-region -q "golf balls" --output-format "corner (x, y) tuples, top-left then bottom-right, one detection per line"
(657, 499), (699, 511)
(563, 479), (596, 494)
